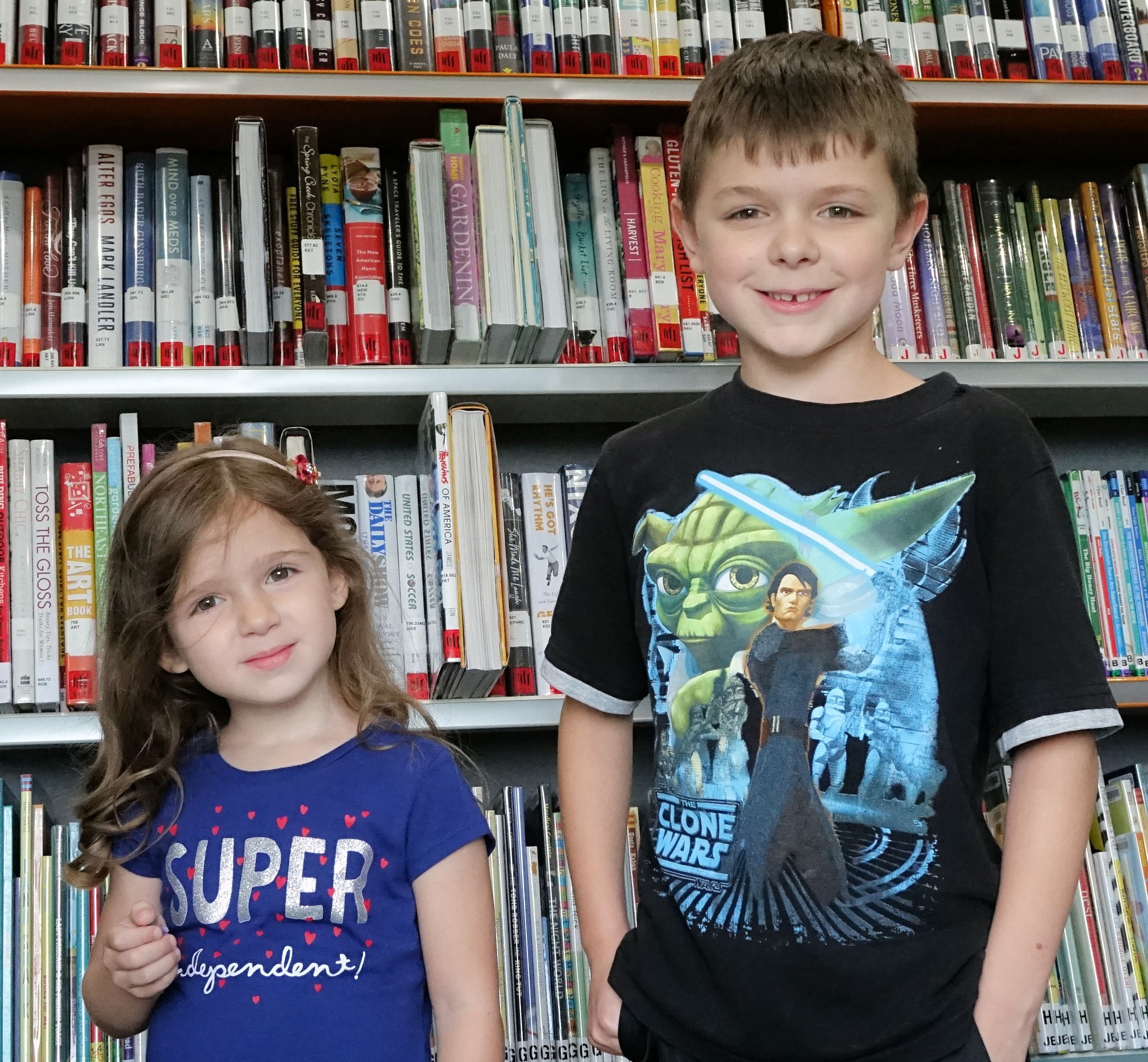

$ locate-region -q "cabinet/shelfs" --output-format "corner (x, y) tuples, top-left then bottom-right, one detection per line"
(0, 0), (1148, 1062)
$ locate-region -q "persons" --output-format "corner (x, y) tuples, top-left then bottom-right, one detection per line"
(68, 434), (511, 1062)
(537, 33), (1125, 1062)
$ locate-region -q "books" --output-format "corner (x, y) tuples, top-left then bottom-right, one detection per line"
(1, 0), (1148, 1062)
